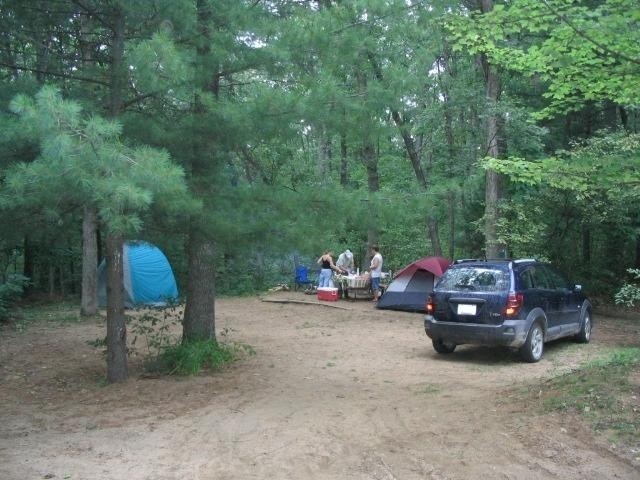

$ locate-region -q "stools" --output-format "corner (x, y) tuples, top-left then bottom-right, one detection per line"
(378, 284), (388, 297)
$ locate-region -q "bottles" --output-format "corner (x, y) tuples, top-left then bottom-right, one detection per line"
(389, 269), (391, 277)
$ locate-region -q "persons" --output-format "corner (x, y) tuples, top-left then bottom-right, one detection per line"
(317, 249), (342, 288)
(368, 244), (383, 302)
(335, 249), (354, 299)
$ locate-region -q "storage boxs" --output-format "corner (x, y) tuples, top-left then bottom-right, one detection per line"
(317, 287), (338, 301)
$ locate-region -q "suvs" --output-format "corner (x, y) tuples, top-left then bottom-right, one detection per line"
(422, 256), (595, 364)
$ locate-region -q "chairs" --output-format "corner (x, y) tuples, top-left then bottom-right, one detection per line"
(295, 264), (315, 291)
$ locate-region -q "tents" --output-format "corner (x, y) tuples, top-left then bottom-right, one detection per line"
(96, 238), (179, 310)
(377, 256), (451, 313)
